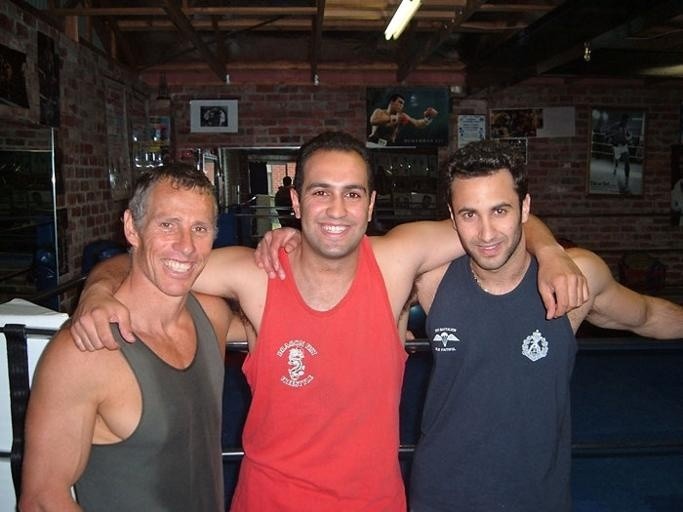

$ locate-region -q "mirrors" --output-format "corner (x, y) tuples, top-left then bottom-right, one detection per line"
(367, 148), (439, 234)
(194, 144), (300, 248)
(0, 120), (62, 315)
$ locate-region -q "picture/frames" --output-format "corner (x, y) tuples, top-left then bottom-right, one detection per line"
(584, 103), (650, 199)
(189, 98), (239, 133)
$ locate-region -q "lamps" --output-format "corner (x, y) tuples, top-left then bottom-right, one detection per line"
(384, 0), (423, 41)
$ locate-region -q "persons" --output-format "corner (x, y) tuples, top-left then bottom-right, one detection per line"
(367, 94), (437, 146)
(610, 112), (631, 185)
(275, 175), (300, 229)
(374, 164), (431, 208)
(19, 166), (240, 512)
(255, 140), (682, 512)
(72, 130), (589, 512)
